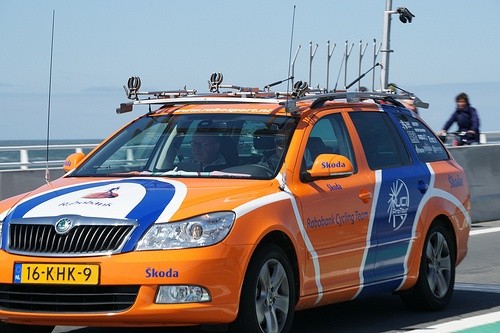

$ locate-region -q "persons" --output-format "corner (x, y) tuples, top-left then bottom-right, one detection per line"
(175, 126), (234, 172)
(252, 128), (316, 176)
(436, 93), (481, 145)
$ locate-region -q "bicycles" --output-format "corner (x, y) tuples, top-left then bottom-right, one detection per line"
(436, 131), (474, 146)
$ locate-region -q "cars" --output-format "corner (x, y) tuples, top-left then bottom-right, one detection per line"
(0, 38), (473, 331)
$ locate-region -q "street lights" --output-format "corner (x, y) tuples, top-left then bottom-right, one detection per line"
(380, 7), (415, 90)
